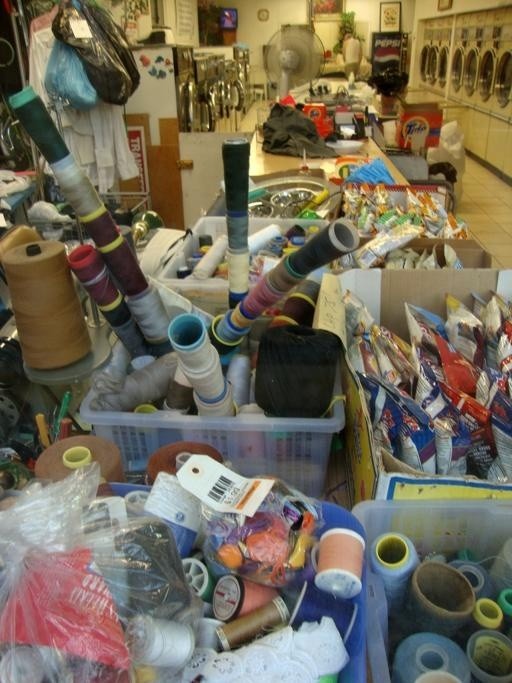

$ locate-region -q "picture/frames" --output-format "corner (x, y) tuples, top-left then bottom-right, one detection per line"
(378, 1), (401, 32)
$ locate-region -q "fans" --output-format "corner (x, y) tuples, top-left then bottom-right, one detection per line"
(263, 24), (327, 102)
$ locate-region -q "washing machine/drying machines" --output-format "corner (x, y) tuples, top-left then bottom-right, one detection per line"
(123, 42), (257, 132)
(411, 1), (511, 185)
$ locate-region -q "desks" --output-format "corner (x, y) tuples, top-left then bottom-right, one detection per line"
(229, 128), (412, 205)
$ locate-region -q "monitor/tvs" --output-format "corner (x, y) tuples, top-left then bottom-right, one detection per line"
(219, 7), (238, 30)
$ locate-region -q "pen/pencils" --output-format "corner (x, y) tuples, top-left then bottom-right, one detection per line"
(11, 391), (73, 472)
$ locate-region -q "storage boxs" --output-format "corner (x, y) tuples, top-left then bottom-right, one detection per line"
(347, 232), (500, 284)
(395, 102), (443, 149)
(350, 495), (507, 675)
(348, 318), (509, 505)
(78, 303), (344, 498)
(79, 473), (363, 676)
(158, 214), (346, 302)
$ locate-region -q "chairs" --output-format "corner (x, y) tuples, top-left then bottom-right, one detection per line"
(387, 154), (457, 213)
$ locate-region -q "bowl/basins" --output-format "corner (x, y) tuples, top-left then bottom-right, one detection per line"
(325, 137), (365, 156)
(245, 189), (316, 219)
